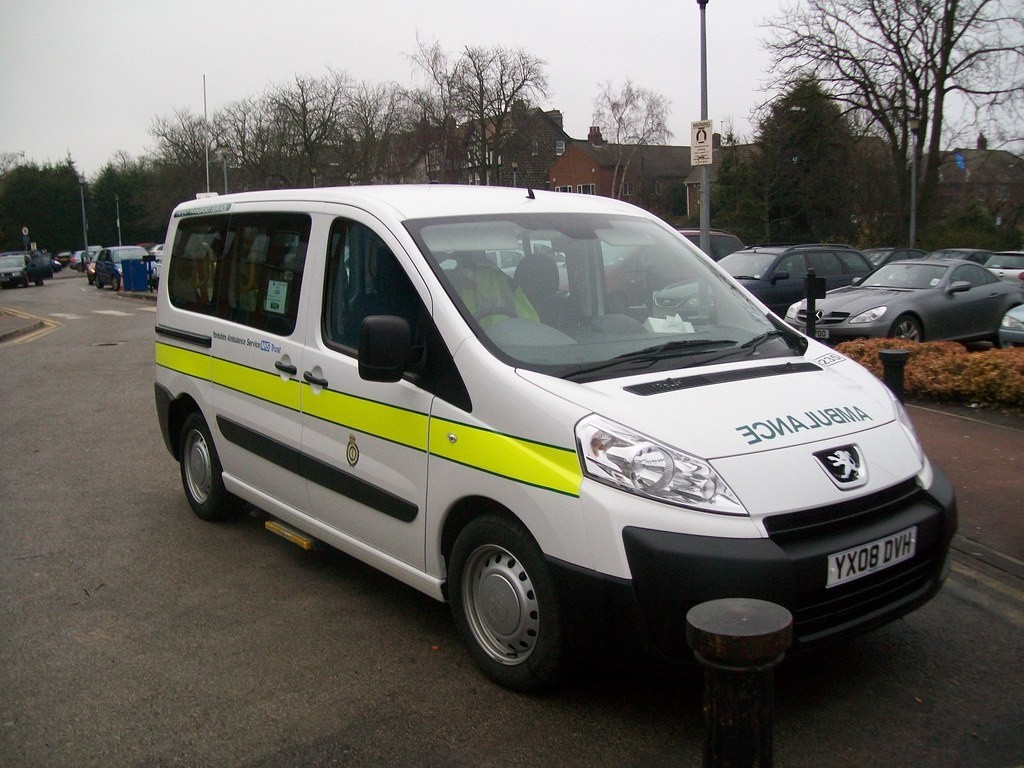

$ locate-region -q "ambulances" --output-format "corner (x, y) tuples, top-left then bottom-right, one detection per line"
(152, 182), (961, 697)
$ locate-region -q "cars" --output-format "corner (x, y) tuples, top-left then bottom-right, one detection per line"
(0, 251), (62, 287)
(925, 248), (993, 261)
(438, 240), (571, 296)
(96, 245), (157, 288)
(862, 247), (931, 271)
(55, 251), (74, 267)
(69, 244), (164, 281)
(783, 260), (1023, 350)
(980, 251), (1024, 286)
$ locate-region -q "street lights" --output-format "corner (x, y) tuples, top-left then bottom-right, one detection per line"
(908, 116), (920, 249)
(115, 194), (122, 246)
(512, 155), (518, 188)
(219, 144), (230, 195)
(311, 165), (317, 188)
(78, 175), (89, 261)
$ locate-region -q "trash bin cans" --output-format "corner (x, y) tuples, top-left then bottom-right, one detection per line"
(121, 258), (147, 292)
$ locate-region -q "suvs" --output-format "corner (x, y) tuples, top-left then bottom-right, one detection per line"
(599, 228), (745, 316)
(654, 243), (878, 325)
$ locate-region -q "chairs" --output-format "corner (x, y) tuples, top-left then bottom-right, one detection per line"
(514, 252), (567, 319)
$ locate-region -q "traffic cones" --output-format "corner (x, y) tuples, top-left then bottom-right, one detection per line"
(119, 274), (126, 292)
